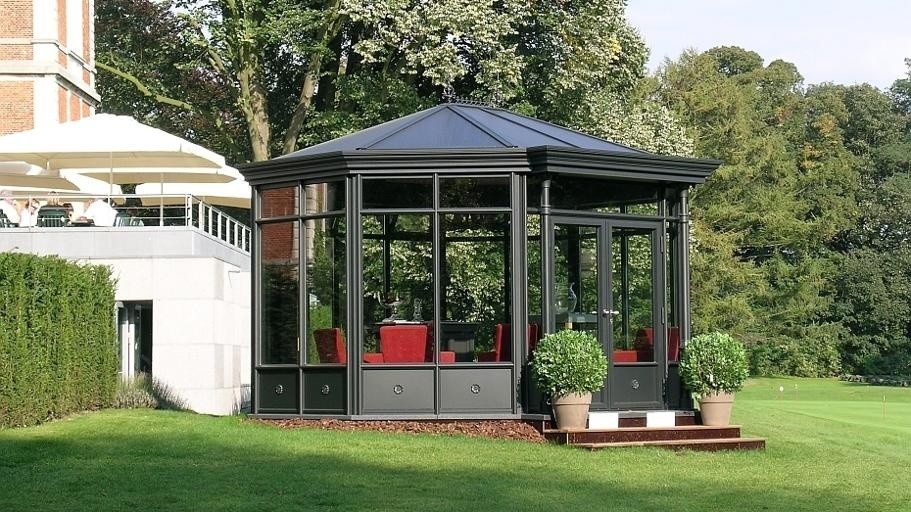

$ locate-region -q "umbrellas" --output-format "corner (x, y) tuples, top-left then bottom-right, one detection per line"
(0, 112), (252, 227)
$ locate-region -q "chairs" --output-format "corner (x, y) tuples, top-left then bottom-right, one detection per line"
(311, 308), (684, 363)
(0, 205), (140, 229)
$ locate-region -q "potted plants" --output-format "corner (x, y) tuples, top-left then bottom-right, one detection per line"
(526, 329), (750, 431)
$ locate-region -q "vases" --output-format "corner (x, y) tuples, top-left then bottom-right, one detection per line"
(411, 298), (424, 323)
(554, 281), (579, 314)
